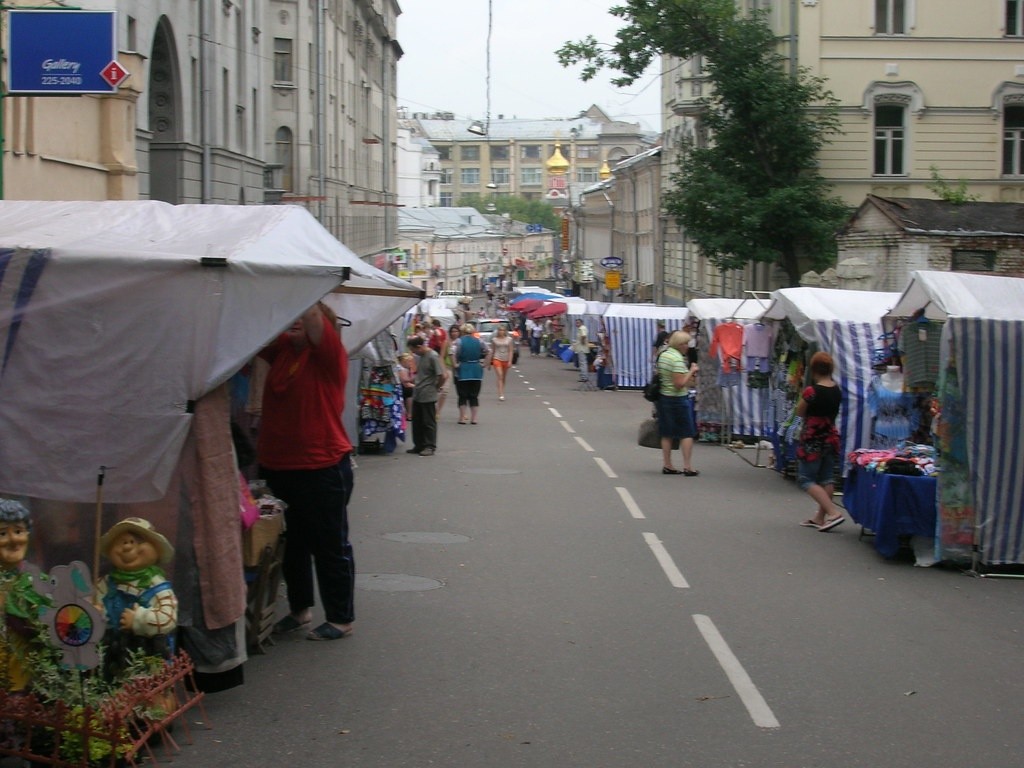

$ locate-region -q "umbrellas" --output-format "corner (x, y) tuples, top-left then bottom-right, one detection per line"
(505, 293), (568, 318)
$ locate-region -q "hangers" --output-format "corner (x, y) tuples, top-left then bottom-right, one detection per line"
(872, 306), (934, 372)
(750, 320), (767, 329)
(718, 317), (739, 329)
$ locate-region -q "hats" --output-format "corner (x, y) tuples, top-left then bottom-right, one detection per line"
(459, 323), (476, 334)
(431, 319), (442, 328)
(651, 331), (671, 346)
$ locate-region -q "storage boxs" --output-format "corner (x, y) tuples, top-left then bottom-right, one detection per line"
(244, 509), (285, 566)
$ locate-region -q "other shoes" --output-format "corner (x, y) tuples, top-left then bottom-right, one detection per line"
(662, 466), (683, 474)
(419, 448), (435, 456)
(406, 445), (422, 454)
(683, 468), (702, 476)
(457, 419), (467, 425)
(499, 396), (504, 400)
(470, 420), (477, 424)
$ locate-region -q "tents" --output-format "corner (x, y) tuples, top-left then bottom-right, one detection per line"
(512, 286), (688, 389)
(0, 203), (421, 693)
(757, 287), (905, 494)
(683, 298), (772, 448)
(881, 269), (1024, 579)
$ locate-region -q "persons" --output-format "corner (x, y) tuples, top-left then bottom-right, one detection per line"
(0, 497), (54, 691)
(397, 282), (566, 425)
(257, 293), (355, 642)
(868, 353), (919, 450)
(574, 320), (589, 383)
(651, 324), (701, 476)
(407, 336), (443, 458)
(93, 518), (177, 667)
(795, 351), (847, 531)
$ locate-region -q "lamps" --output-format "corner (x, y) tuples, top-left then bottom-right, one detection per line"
(485, 181), (498, 189)
(485, 203), (497, 212)
(467, 120), (486, 136)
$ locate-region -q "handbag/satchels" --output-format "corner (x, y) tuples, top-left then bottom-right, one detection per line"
(638, 417), (681, 450)
(643, 372), (660, 402)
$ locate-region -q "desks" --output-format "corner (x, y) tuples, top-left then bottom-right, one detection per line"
(842, 465), (936, 556)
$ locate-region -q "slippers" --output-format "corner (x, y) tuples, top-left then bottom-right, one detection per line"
(799, 520), (825, 529)
(274, 611), (316, 633)
(305, 622), (353, 640)
(818, 513), (845, 532)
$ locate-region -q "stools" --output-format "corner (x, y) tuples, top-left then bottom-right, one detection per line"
(576, 371), (598, 392)
(245, 558), (285, 656)
